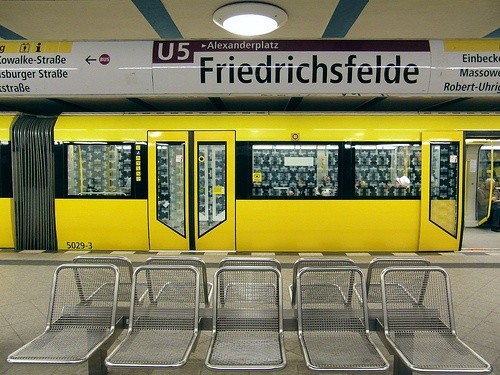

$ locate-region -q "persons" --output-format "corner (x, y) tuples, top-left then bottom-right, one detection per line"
(118, 152), (131, 188)
(86, 177), (98, 192)
(480, 170), (500, 182)
(476, 178), (500, 233)
(253, 154), (421, 197)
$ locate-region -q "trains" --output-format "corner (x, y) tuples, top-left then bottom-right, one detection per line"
(0, 110), (500, 252)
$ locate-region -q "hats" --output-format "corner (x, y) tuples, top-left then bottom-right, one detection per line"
(395, 176), (411, 188)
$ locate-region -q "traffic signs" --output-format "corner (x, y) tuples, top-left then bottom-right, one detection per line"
(0, 40), (153, 97)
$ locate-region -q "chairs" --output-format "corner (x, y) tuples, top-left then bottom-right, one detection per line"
(5, 253), (494, 373)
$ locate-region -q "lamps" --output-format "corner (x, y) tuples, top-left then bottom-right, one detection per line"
(210, 0), (291, 38)
